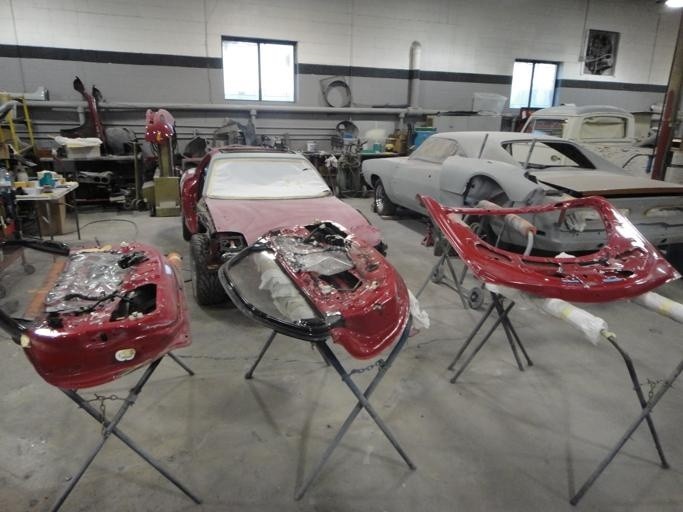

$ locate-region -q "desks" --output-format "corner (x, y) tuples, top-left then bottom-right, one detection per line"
(16, 179), (78, 235)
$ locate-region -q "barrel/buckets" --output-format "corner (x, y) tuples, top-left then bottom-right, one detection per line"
(307, 141), (319, 152)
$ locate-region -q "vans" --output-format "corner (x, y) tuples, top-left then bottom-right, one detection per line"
(520, 105), (636, 146)
